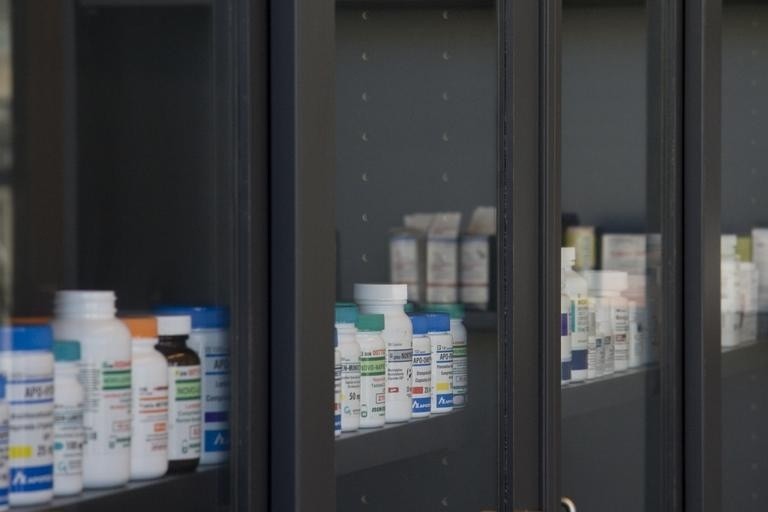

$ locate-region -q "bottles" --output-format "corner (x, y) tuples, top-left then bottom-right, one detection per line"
(0, 323), (53, 507)
(333, 282), (469, 434)
(155, 313), (203, 474)
(0, 374), (9, 512)
(561, 245), (640, 387)
(53, 289), (131, 489)
(117, 314), (168, 482)
(719, 252), (759, 349)
(53, 339), (84, 498)
(157, 303), (230, 465)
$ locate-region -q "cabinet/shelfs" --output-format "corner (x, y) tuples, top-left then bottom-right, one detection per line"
(1, 0), (768, 512)
